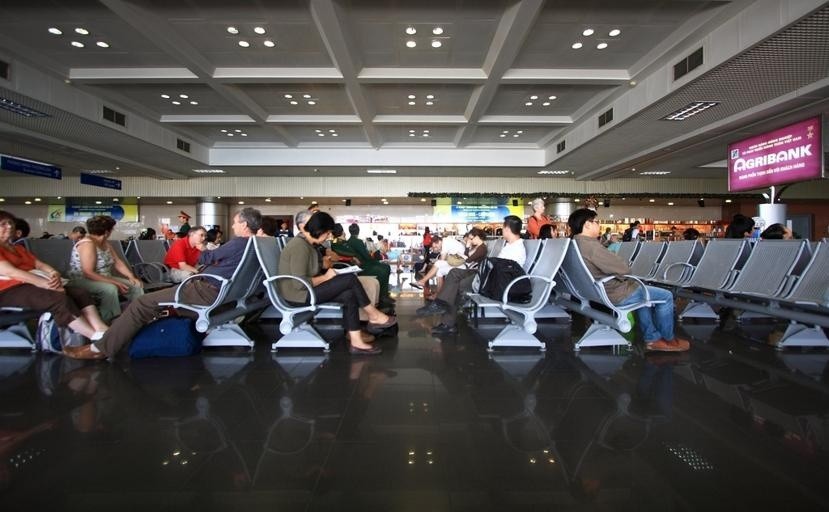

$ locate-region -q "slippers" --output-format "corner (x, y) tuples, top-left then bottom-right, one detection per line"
(409, 282), (424, 289)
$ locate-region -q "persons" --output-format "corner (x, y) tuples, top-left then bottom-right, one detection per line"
(63, 226), (87, 245)
(417, 215), (528, 335)
(256, 203), (398, 356)
(62, 205), (262, 363)
(40, 232), (53, 240)
(400, 213), (799, 315)
(9, 218), (29, 245)
(302, 356), (398, 494)
(0, 210), (111, 342)
(527, 198), (552, 239)
(67, 214), (146, 325)
(139, 211), (225, 284)
(569, 208), (691, 354)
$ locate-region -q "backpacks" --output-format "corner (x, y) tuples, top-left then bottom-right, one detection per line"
(471, 257), (533, 304)
(32, 309), (86, 356)
(623, 227), (633, 242)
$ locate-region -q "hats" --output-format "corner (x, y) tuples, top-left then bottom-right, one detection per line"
(177, 209), (191, 220)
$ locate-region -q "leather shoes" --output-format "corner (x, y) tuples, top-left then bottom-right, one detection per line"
(62, 345), (108, 360)
(366, 315), (398, 329)
(343, 329), (376, 342)
(349, 344), (383, 356)
(647, 335), (689, 353)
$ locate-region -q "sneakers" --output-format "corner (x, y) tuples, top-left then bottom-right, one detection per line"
(380, 295), (395, 304)
(417, 303), (448, 315)
(432, 323), (458, 334)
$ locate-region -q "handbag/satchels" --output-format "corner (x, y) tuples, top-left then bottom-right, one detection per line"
(446, 253), (465, 267)
